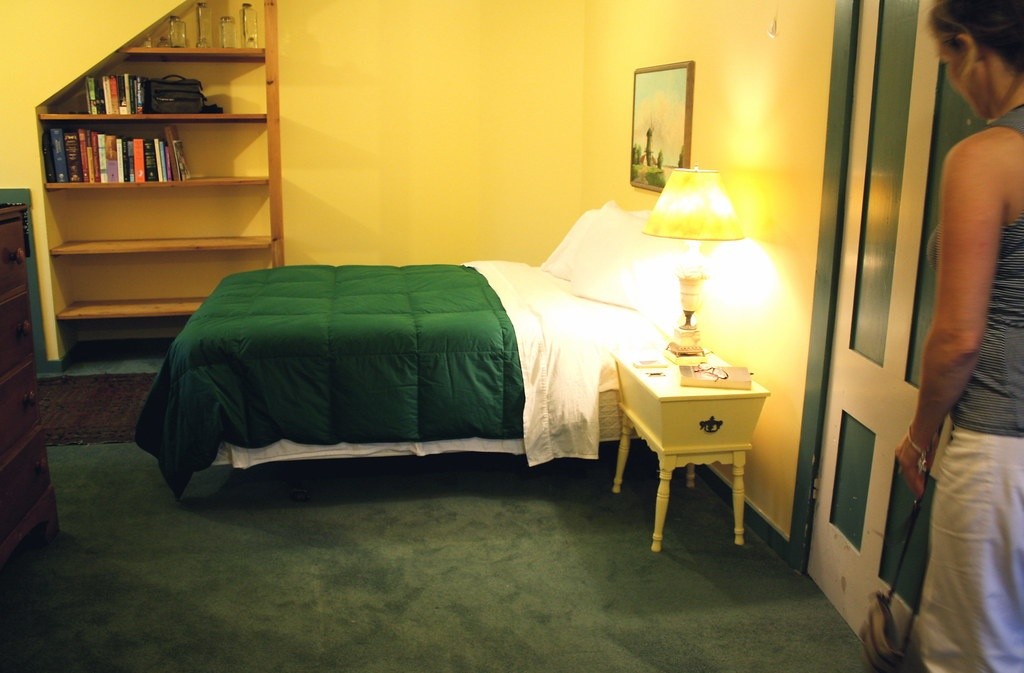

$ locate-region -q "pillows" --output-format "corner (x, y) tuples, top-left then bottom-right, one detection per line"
(575, 201), (650, 310)
(540, 209), (647, 281)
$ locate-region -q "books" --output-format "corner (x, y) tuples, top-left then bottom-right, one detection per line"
(678, 365), (752, 391)
(42, 125), (190, 183)
(84, 76), (148, 114)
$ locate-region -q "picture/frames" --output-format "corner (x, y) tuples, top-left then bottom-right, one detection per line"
(630, 60), (696, 192)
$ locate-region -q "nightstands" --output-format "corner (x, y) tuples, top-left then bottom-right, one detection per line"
(611, 353), (771, 551)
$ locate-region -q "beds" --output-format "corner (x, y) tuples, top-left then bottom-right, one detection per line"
(134, 260), (665, 502)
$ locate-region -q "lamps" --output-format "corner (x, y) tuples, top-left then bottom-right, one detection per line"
(642, 169), (744, 363)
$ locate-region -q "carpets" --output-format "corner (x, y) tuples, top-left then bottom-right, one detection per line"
(36, 373), (157, 446)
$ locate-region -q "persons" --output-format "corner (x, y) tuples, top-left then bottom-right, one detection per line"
(894, 0), (1024, 673)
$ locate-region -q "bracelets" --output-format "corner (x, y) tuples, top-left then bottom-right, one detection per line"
(908, 423), (938, 473)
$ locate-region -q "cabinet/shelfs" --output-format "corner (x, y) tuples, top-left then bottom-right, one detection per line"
(41, 0), (285, 362)
(0, 205), (59, 606)
